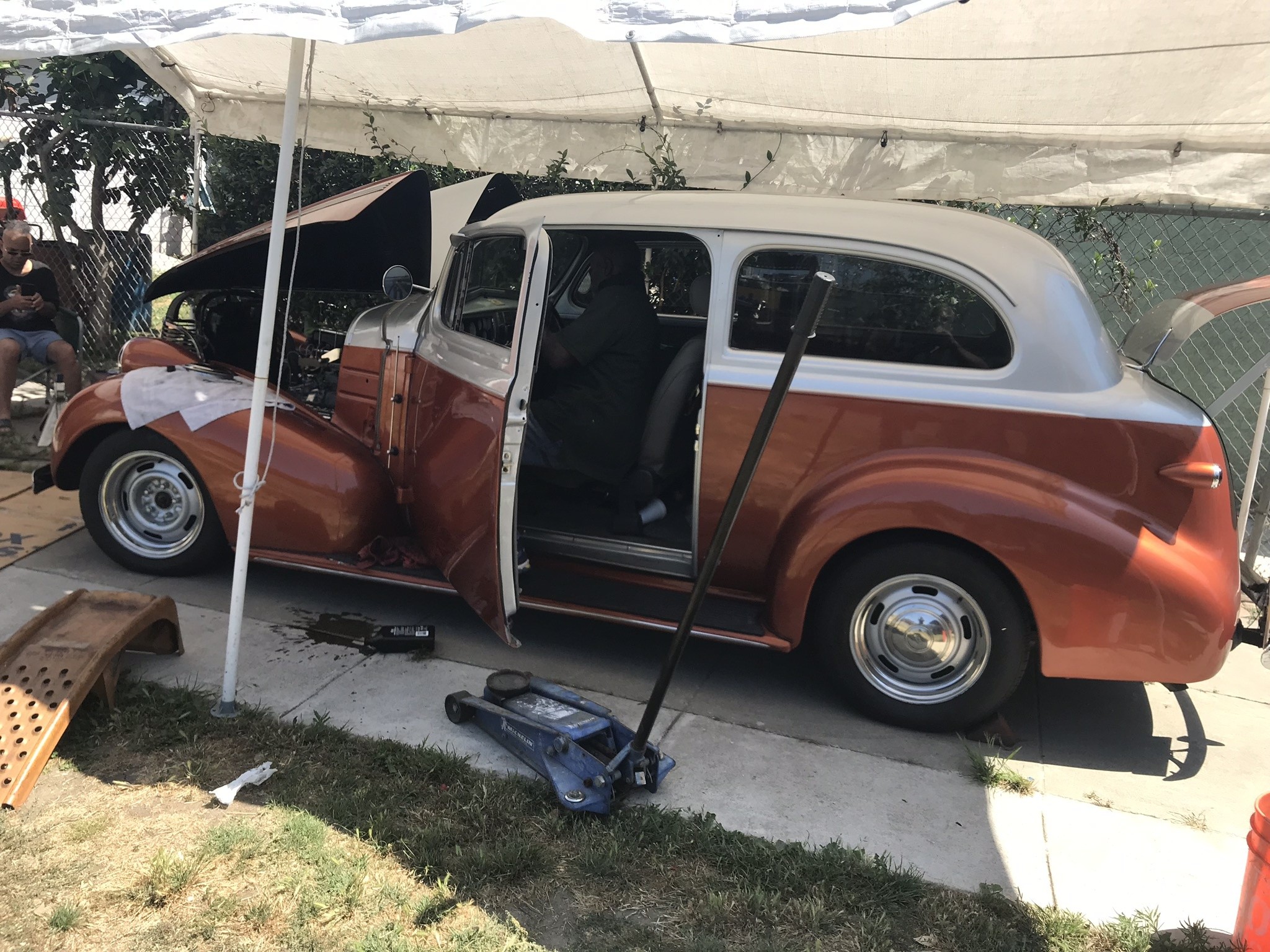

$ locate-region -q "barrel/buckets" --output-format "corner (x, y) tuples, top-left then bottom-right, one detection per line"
(1232, 791), (1270, 952)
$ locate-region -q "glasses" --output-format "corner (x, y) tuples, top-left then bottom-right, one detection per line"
(3, 247), (34, 258)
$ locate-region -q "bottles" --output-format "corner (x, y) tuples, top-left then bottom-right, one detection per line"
(364, 625), (436, 652)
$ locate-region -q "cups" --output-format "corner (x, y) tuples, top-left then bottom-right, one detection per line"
(639, 498), (667, 525)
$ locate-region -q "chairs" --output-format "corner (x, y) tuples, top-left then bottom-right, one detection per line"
(519, 274), (711, 536)
(15, 307), (83, 404)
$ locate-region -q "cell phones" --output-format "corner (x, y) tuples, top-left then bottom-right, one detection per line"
(22, 283), (34, 297)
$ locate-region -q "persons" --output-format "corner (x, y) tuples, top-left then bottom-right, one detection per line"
(0, 220), (82, 434)
(514, 240), (660, 488)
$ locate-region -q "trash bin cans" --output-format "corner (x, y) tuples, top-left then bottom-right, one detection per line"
(79, 228), (152, 331)
(0, 197), (26, 254)
(32, 241), (80, 349)
(1232, 793), (1270, 952)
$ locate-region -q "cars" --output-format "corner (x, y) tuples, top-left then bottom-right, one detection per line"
(31, 157), (1269, 737)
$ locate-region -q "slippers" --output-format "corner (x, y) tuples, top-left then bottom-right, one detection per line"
(0, 419), (14, 436)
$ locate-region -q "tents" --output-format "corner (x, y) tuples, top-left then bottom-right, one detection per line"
(0, 0), (1269, 727)
